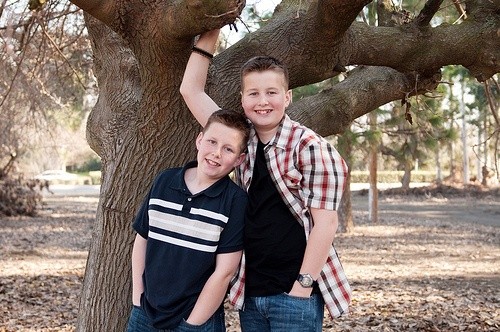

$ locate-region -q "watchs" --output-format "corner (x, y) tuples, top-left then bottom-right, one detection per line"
(297, 274), (317, 288)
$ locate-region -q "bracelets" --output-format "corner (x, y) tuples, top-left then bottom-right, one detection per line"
(190, 46), (214, 60)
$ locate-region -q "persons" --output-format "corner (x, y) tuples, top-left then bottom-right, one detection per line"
(127, 110), (250, 332)
(180, 30), (352, 331)
